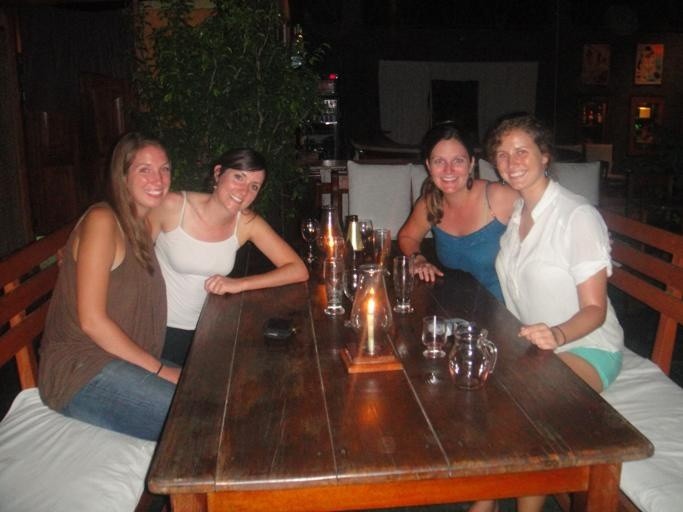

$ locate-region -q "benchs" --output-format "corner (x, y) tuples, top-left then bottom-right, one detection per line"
(0, 217), (169, 512)
(552, 206), (683, 512)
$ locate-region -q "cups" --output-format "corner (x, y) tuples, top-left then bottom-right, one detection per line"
(299, 203), (415, 364)
(420, 314), (451, 359)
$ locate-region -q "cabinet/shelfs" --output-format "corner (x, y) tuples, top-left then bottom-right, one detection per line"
(307, 79), (344, 160)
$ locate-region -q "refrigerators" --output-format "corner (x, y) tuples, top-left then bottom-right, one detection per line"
(304, 75), (349, 164)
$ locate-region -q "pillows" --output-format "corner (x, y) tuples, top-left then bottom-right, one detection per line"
(347, 158), (601, 241)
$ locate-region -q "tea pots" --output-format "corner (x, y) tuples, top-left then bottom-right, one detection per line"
(448, 321), (499, 391)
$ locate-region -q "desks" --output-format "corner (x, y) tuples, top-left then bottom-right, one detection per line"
(308, 144), (613, 241)
(146, 239), (654, 512)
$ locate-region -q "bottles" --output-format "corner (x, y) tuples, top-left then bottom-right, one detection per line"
(634, 106), (656, 150)
(579, 102), (604, 124)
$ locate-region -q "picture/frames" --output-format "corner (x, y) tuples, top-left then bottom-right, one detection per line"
(626, 95), (664, 157)
(576, 96), (616, 146)
(581, 40), (611, 88)
(632, 39), (665, 88)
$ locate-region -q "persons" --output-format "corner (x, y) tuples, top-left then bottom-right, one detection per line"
(395, 125), (520, 309)
(36, 130), (183, 440)
(482, 111), (626, 396)
(146, 144), (309, 369)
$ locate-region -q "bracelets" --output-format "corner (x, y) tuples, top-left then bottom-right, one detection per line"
(551, 325), (566, 346)
(156, 362), (163, 376)
(408, 252), (423, 260)
